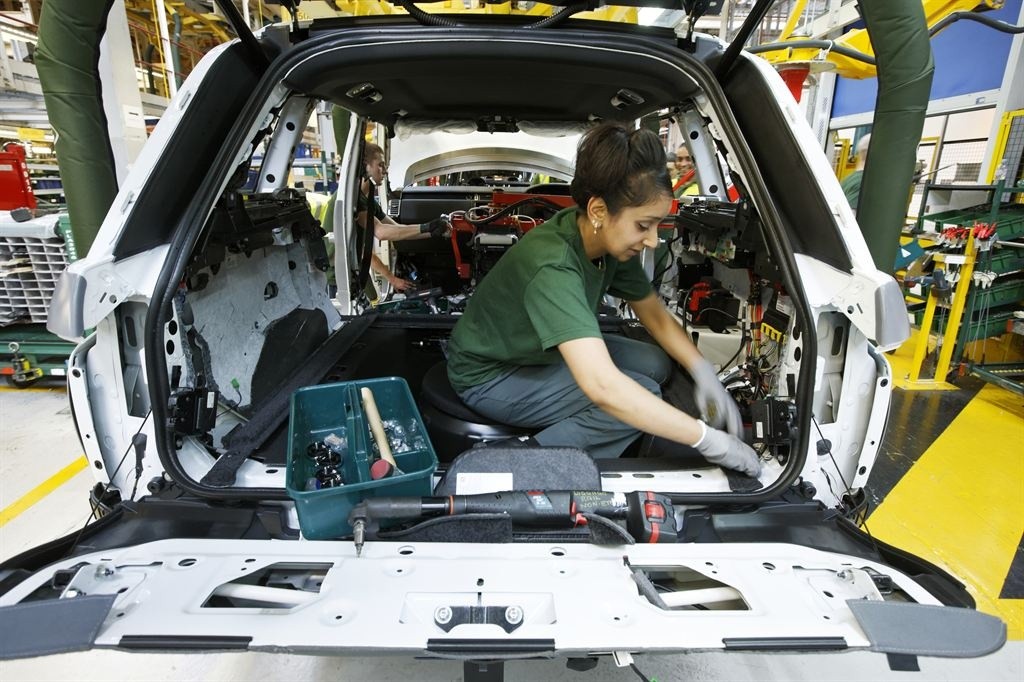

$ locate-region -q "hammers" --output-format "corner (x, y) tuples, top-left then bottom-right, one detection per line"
(360, 388), (406, 479)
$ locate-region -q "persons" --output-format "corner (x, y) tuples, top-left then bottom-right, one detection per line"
(448, 124), (760, 478)
(841, 133), (871, 217)
(323, 142), (447, 301)
(670, 142), (699, 199)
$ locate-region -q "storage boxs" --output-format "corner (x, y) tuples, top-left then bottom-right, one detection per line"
(286, 377), (439, 540)
(904, 201), (1024, 341)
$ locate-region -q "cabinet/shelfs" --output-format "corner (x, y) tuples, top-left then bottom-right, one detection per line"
(0, 237), (70, 322)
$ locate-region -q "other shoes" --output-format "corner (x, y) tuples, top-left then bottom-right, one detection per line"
(473, 437), (526, 451)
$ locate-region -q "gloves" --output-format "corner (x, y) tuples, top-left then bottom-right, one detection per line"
(687, 358), (744, 439)
(690, 420), (762, 479)
(419, 220), (453, 239)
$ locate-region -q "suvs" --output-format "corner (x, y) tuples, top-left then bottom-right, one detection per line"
(1, 0), (1009, 682)
